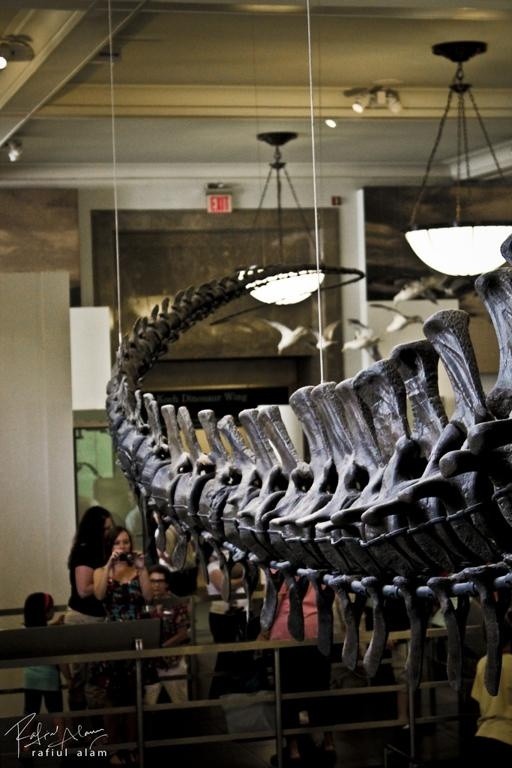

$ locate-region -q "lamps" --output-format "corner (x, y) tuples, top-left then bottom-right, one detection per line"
(234, 39), (512, 306)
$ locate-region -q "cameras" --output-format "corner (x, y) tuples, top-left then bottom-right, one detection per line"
(118, 551), (135, 566)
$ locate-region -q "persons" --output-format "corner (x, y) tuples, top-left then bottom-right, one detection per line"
(21, 506), (511, 768)
(124, 491), (142, 552)
